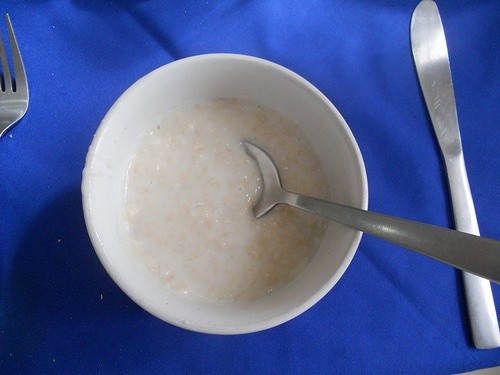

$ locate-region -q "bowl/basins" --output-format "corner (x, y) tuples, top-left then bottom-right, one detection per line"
(80, 53), (369, 334)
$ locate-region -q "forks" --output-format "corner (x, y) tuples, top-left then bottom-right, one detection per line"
(0, 11), (30, 136)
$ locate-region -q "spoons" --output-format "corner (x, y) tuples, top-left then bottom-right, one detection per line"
(242, 140), (499, 285)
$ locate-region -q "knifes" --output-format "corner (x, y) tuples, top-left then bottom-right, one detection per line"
(409, 0), (500, 350)
